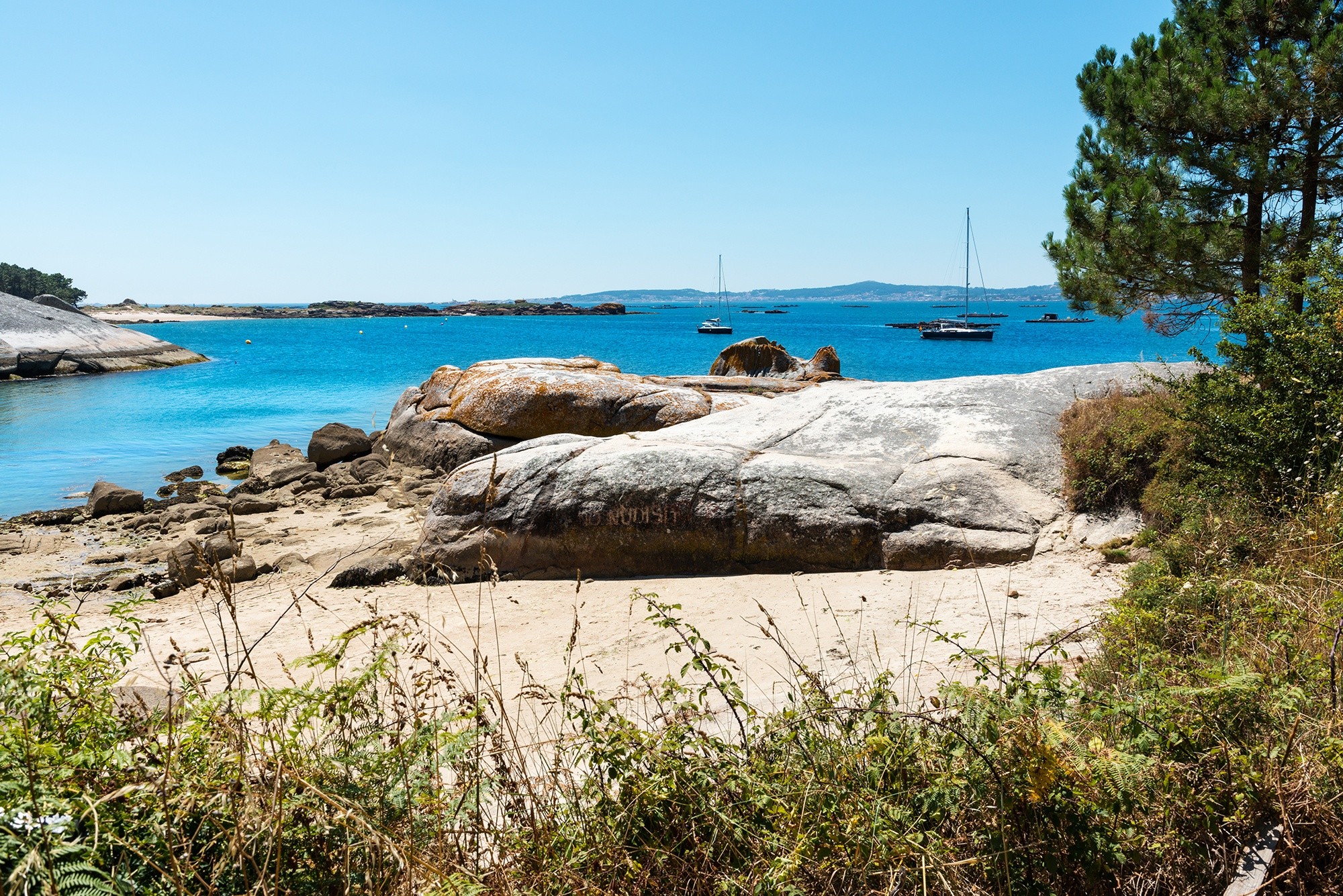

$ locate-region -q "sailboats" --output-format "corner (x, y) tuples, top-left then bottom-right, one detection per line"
(696, 253), (733, 334)
(921, 207), (995, 342)
